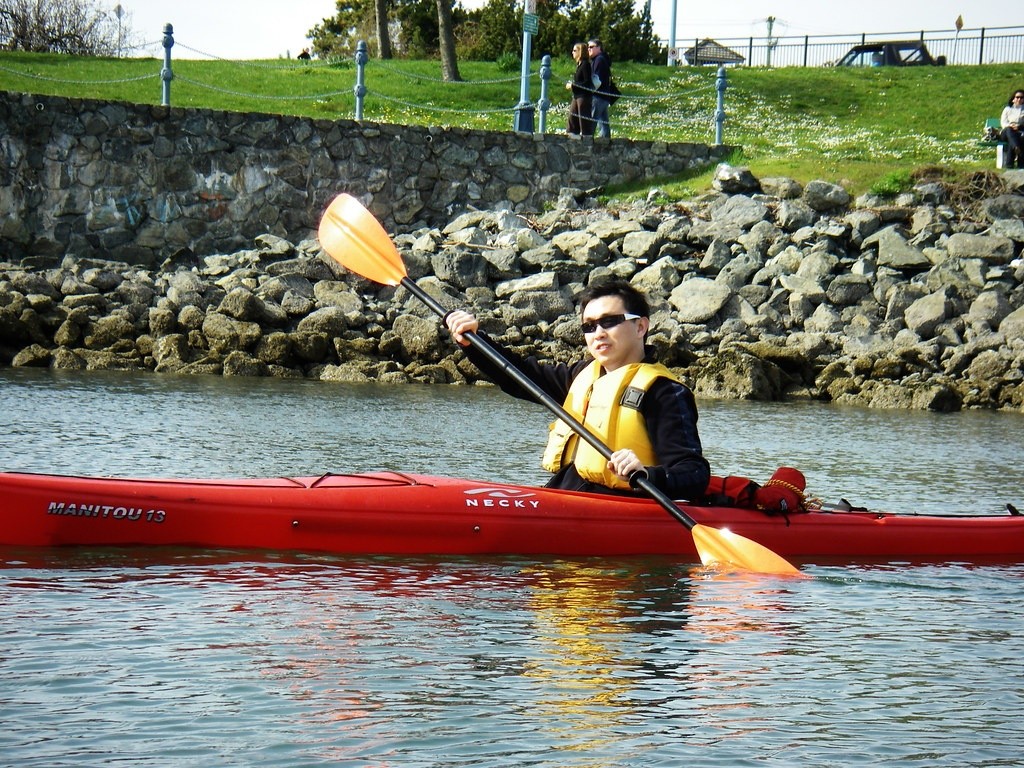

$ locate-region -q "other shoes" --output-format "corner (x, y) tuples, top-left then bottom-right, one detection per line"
(1006, 165), (1014, 169)
(1014, 146), (1021, 156)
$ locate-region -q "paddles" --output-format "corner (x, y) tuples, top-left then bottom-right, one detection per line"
(313, 191), (802, 575)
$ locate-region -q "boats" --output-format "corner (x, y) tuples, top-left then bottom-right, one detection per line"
(0, 469), (1024, 569)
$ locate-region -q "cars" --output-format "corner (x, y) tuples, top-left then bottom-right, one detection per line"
(835, 40), (947, 69)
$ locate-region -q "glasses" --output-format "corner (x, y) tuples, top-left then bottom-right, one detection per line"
(1015, 96), (1024, 100)
(587, 45), (599, 49)
(580, 313), (642, 333)
(573, 50), (580, 53)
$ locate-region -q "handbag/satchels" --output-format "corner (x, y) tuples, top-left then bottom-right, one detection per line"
(589, 73), (602, 93)
(609, 82), (622, 107)
(981, 126), (999, 143)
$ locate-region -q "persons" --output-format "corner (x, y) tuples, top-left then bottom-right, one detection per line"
(565, 42), (594, 141)
(871, 53), (883, 66)
(587, 39), (613, 139)
(298, 48), (311, 59)
(999, 89), (1024, 169)
(446, 281), (710, 502)
